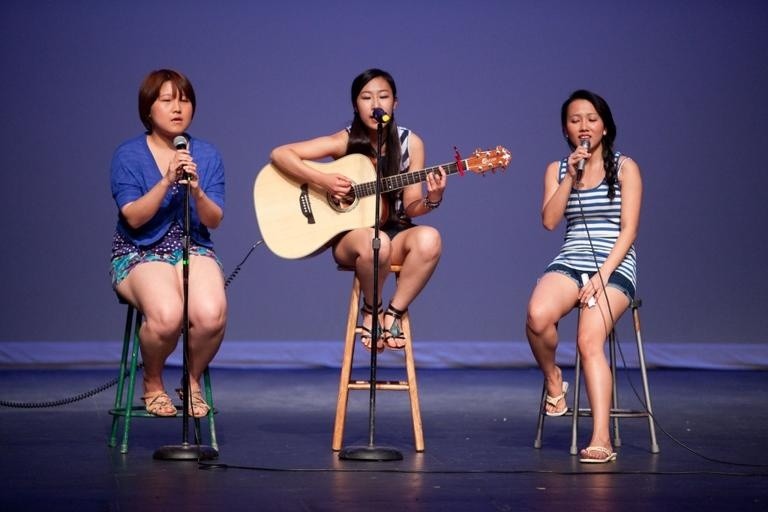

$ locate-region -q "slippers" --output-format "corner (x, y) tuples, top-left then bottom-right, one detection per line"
(580, 447), (617, 463)
(544, 382), (569, 415)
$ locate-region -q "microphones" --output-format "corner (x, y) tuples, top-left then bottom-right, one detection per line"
(372, 107), (391, 124)
(575, 137), (591, 182)
(172, 134), (192, 183)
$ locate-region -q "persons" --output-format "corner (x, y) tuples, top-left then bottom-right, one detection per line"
(526, 89), (642, 462)
(110, 69), (227, 418)
(271, 68), (447, 354)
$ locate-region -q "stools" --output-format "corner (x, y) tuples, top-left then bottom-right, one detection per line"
(332, 264), (424, 452)
(109, 290), (218, 453)
(535, 296), (658, 453)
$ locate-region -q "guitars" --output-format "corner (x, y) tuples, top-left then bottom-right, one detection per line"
(253, 145), (513, 261)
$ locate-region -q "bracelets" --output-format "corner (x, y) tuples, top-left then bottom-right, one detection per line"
(423, 194), (443, 210)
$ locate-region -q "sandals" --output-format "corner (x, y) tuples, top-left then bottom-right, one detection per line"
(384, 300), (408, 350)
(360, 296), (384, 353)
(180, 390), (210, 417)
(141, 391), (178, 416)
